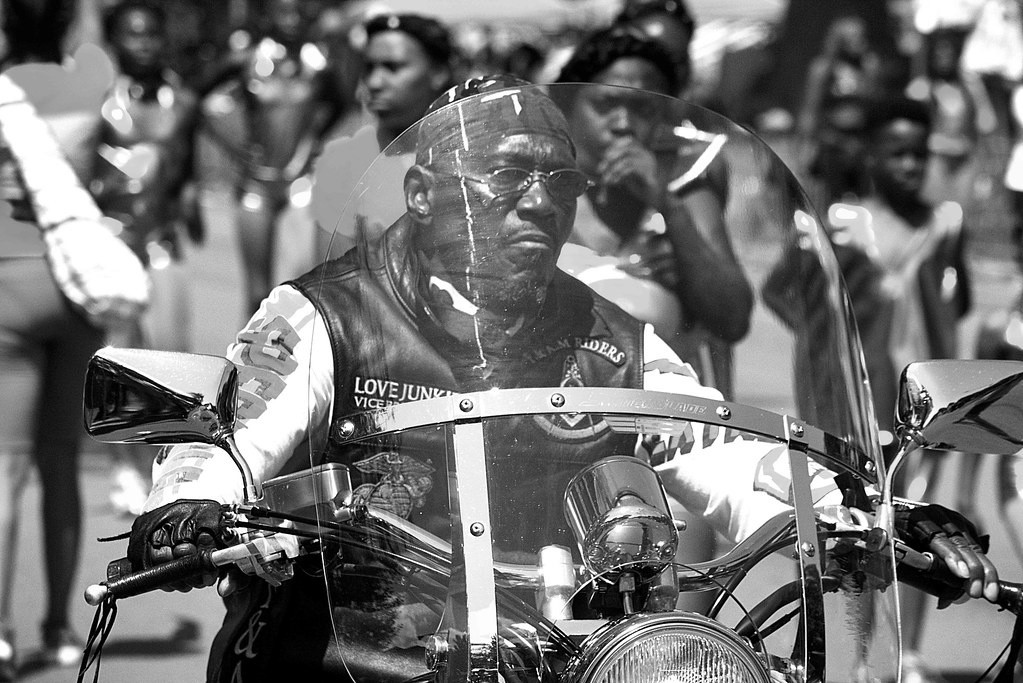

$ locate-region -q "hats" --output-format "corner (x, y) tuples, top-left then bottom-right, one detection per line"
(611, 0), (695, 41)
(365, 14), (450, 62)
(546, 29), (681, 115)
(414, 72), (579, 166)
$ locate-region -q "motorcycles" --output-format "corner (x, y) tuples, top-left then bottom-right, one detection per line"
(80, 78), (1021, 683)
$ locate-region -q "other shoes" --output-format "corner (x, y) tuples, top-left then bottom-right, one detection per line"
(39, 624), (86, 664)
(0, 627), (17, 679)
(849, 667), (871, 683)
(894, 650), (930, 682)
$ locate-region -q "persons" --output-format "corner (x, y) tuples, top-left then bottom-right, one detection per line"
(198, 0), (348, 308)
(548, 30), (752, 345)
(315, 10), (452, 262)
(799, 0), (1022, 218)
(609, 2), (696, 92)
(763, 96), (974, 681)
(126, 74), (1000, 681)
(103, 2), (198, 513)
(0, 0), (105, 680)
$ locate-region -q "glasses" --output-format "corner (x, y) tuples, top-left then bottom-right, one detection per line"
(431, 166), (593, 202)
